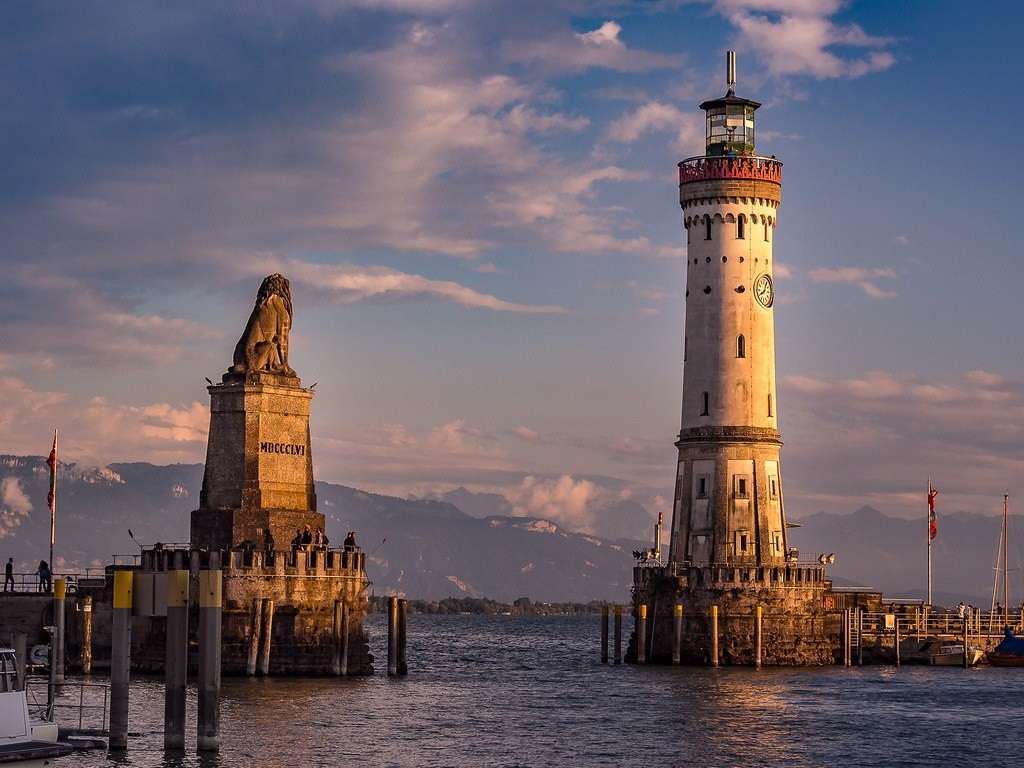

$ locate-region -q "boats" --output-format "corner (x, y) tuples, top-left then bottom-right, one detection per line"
(930, 645), (983, 666)
(985, 625), (1024, 666)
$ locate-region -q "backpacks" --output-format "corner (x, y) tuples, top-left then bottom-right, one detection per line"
(323, 535), (329, 545)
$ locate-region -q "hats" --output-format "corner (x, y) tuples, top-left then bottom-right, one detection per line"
(306, 525), (310, 530)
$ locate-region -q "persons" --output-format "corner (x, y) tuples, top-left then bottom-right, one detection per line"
(262, 522), (275, 558)
(291, 524), (328, 550)
(888, 601), (896, 613)
(994, 601), (1005, 632)
(955, 602), (973, 619)
(36, 560), (56, 594)
(345, 532), (359, 550)
(917, 601), (927, 632)
(4, 557), (20, 593)
